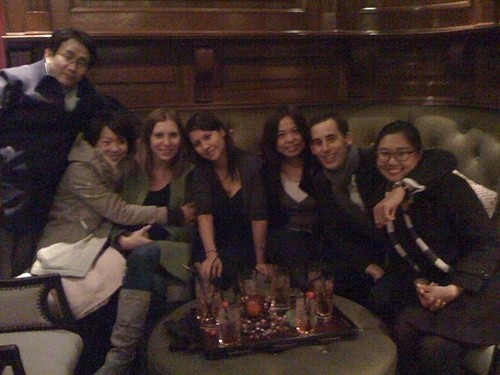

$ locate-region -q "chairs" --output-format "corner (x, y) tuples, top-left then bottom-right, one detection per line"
(0, 275), (96, 375)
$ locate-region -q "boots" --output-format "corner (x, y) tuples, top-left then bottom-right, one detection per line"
(165, 277), (191, 310)
(91, 287), (152, 374)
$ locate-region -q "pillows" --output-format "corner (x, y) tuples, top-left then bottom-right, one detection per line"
(451, 169), (498, 219)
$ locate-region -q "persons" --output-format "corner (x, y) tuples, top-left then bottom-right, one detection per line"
(184, 108), (268, 299)
(31, 109), (197, 375)
(1, 29), (103, 278)
(260, 105), (318, 299)
(308, 110), (458, 312)
(93, 106), (197, 374)
(356, 119), (500, 375)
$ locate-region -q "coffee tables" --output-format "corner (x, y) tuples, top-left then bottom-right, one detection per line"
(148, 288), (396, 375)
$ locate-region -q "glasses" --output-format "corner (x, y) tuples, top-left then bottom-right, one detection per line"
(54, 52), (91, 68)
(376, 148), (419, 160)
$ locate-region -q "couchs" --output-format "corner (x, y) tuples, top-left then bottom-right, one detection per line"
(0, 104), (500, 375)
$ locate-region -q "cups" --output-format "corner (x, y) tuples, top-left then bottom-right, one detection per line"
(194, 277), (223, 329)
(315, 277), (334, 322)
(296, 290), (317, 332)
(217, 309), (240, 345)
(271, 271), (291, 311)
(243, 275), (262, 317)
